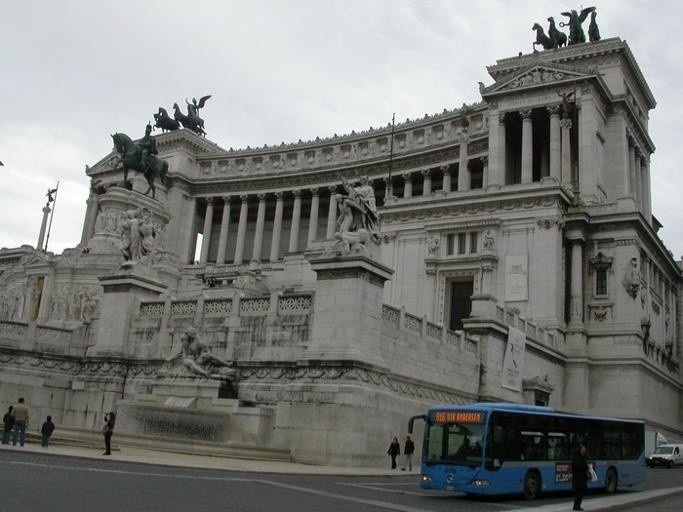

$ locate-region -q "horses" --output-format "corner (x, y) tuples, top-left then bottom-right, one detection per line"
(532, 10), (600, 53)
(152, 101), (204, 134)
(110, 132), (169, 199)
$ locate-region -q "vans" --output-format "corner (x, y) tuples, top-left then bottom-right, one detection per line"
(648, 441), (683, 468)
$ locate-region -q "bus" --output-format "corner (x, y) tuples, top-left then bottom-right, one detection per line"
(403, 401), (648, 505)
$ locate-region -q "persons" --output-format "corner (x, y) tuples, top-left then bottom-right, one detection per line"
(101, 411), (115, 455)
(570, 443), (589, 511)
(41, 415), (56, 448)
(336, 175), (381, 237)
(387, 436), (401, 469)
(400, 435), (415, 471)
(139, 121), (159, 170)
(334, 193), (367, 232)
(2, 405), (15, 445)
(10, 397), (30, 447)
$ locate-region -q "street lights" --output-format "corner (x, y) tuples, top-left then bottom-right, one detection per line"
(0, 160), (5, 168)
